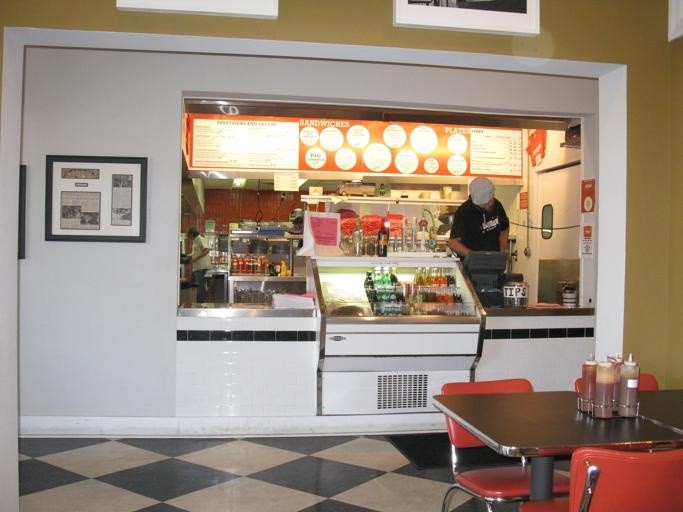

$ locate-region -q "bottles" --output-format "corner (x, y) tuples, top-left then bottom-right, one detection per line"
(379, 182), (385, 197)
(581, 352), (640, 418)
(377, 218), (388, 257)
(231, 254), (257, 273)
(367, 226), (375, 256)
(364, 266), (463, 316)
(352, 216), (364, 257)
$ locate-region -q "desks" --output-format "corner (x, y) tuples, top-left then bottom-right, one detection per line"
(432, 389), (682, 500)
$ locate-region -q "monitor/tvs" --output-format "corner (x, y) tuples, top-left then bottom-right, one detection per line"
(462, 251), (508, 280)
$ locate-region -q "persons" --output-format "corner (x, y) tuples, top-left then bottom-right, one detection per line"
(186, 227), (210, 303)
(448, 178), (509, 260)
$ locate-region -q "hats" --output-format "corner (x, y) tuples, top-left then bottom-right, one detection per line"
(467, 177), (497, 206)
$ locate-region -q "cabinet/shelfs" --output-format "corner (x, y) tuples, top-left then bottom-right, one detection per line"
(227, 233), (517, 317)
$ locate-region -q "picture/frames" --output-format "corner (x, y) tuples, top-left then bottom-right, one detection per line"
(44, 154), (148, 243)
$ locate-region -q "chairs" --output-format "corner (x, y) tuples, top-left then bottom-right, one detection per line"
(442, 374), (683, 511)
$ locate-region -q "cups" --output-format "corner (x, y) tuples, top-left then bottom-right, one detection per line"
(442, 186), (452, 200)
